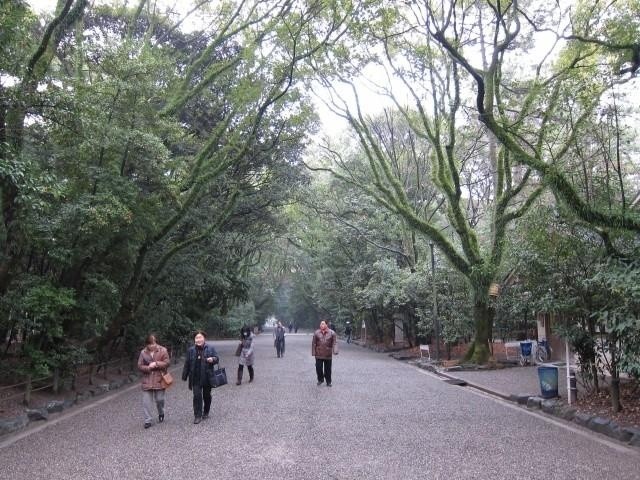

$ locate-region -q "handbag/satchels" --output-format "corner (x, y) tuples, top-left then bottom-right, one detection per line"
(206, 367), (228, 387)
(235, 344), (244, 356)
(160, 373), (173, 388)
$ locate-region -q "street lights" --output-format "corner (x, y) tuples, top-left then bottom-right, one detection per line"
(428, 235), (440, 363)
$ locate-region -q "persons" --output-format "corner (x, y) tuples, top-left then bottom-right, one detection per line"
(137, 334), (171, 429)
(294, 324), (297, 333)
(181, 329), (220, 424)
(311, 319), (340, 387)
(288, 323), (292, 333)
(234, 325), (257, 387)
(272, 321), (287, 359)
(344, 323), (353, 345)
(329, 322), (338, 345)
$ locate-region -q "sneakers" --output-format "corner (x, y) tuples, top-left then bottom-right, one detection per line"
(159, 414), (164, 423)
(316, 380), (333, 387)
(144, 423), (151, 428)
(194, 412), (209, 425)
(235, 377), (254, 385)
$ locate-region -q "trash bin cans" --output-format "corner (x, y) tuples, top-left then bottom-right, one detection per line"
(538, 368), (558, 397)
